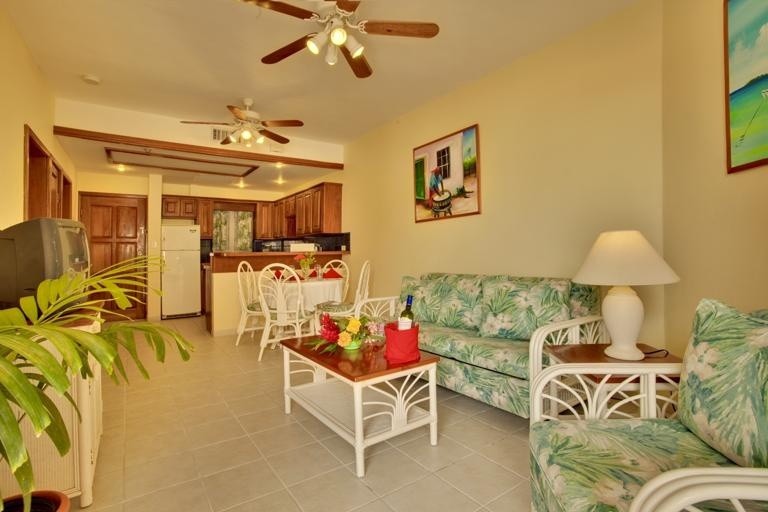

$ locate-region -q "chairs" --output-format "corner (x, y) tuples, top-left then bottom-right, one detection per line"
(322, 259), (349, 304)
(256, 263), (317, 362)
(235, 258), (277, 347)
(315, 260), (372, 335)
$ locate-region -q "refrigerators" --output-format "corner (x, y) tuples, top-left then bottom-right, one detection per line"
(161, 224), (202, 319)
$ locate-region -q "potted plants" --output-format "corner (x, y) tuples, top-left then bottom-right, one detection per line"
(0, 252), (198, 512)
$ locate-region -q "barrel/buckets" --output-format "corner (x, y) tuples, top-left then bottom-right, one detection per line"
(383, 320), (420, 364)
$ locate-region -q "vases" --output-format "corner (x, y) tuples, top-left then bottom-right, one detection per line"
(299, 262), (310, 280)
(341, 340), (360, 350)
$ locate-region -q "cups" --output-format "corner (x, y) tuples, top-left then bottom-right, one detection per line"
(289, 264), (295, 271)
(341, 245), (346, 251)
(316, 266), (323, 280)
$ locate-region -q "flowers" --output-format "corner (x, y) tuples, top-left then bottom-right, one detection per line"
(304, 310), (387, 359)
(292, 247), (316, 281)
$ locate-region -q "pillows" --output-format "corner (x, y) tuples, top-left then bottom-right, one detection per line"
(478, 279), (573, 345)
(673, 294), (768, 471)
(394, 273), (488, 332)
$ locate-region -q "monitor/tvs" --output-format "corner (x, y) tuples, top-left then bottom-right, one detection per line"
(0, 217), (90, 315)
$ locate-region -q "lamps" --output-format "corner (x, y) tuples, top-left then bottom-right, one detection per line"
(228, 124), (266, 148)
(304, 20), (364, 69)
(571, 229), (684, 360)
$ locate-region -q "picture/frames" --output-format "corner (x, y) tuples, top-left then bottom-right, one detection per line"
(412, 123), (482, 223)
(723, 1), (768, 175)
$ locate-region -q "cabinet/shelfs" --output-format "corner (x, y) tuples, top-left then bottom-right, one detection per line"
(273, 201), (296, 239)
(254, 201), (273, 239)
(296, 192), (305, 236)
(197, 199), (214, 239)
(162, 196), (197, 219)
(305, 189), (312, 234)
(0, 298), (107, 507)
(285, 196), (296, 217)
(313, 181), (343, 232)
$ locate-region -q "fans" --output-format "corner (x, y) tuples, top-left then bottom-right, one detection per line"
(241, 1), (441, 82)
(177, 98), (306, 146)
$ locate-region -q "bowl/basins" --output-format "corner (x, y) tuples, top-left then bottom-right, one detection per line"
(431, 191), (452, 207)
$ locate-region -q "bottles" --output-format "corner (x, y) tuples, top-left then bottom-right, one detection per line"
(398, 295), (413, 331)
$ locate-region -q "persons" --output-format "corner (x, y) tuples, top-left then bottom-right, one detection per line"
(428, 166), (444, 208)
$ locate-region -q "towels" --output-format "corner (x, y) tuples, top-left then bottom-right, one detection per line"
(309, 269), (318, 276)
(272, 267), (283, 279)
(322, 267), (342, 278)
(289, 273), (306, 282)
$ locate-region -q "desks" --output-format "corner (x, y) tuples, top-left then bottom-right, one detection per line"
(544, 343), (684, 426)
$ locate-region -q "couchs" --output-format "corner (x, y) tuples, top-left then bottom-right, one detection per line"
(526, 298), (768, 512)
(359, 269), (598, 424)
